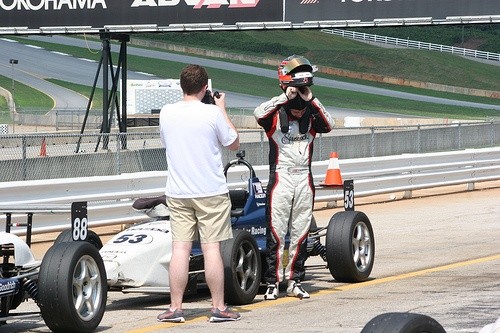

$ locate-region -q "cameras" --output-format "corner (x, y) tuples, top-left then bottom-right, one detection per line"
(200, 90), (219, 105)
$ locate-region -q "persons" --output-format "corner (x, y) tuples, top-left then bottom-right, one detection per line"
(253, 85), (336, 299)
(157, 63), (241, 323)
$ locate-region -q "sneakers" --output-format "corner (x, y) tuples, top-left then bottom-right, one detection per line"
(263, 281), (280, 299)
(210, 307), (241, 322)
(287, 280), (310, 298)
(156, 306), (185, 323)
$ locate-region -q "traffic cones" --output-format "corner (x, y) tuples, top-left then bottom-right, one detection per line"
(37, 138), (48, 157)
(318, 151), (344, 187)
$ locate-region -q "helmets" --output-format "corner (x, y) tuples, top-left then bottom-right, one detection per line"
(278, 55), (314, 92)
